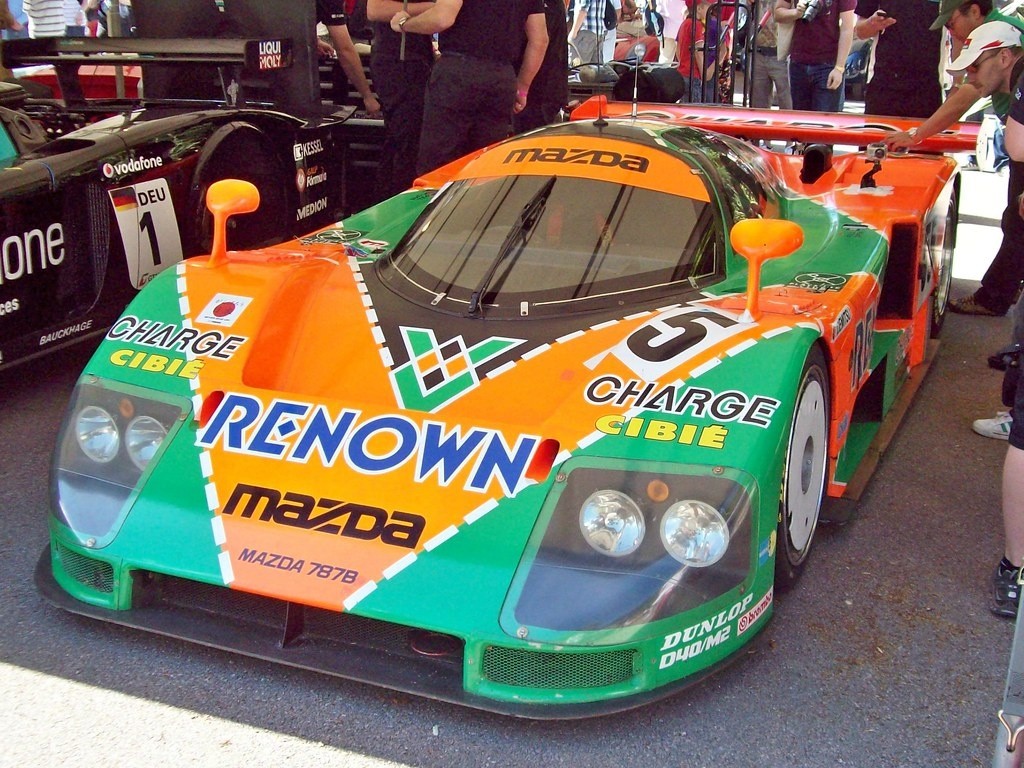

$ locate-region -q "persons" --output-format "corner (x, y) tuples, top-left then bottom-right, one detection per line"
(884, 0), (1024, 619)
(316, 0), (568, 206)
(563, 0), (943, 118)
(0, 0), (134, 82)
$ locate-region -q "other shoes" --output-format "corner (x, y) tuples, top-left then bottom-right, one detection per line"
(987, 351), (1022, 370)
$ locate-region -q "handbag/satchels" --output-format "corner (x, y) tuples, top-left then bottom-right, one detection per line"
(645, 7), (664, 37)
(604, 0), (617, 31)
(612, 60), (685, 103)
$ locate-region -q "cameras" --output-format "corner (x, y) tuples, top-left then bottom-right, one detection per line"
(800, 0), (822, 25)
(867, 143), (888, 160)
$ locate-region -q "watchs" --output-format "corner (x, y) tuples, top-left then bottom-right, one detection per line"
(907, 128), (922, 144)
(399, 17), (410, 32)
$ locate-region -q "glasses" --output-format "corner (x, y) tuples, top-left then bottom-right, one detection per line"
(965, 53), (1001, 74)
(945, 8), (970, 31)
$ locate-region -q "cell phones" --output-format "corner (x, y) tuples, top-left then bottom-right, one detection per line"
(876, 12), (887, 18)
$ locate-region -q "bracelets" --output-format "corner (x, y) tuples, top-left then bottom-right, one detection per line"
(519, 91), (528, 97)
(836, 65), (844, 73)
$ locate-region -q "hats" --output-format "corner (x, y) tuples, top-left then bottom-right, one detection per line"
(930, 0), (966, 31)
(946, 21), (1021, 77)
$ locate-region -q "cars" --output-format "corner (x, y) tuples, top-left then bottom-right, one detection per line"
(844, 38), (878, 101)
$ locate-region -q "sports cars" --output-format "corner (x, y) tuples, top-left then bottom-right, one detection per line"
(31, 93), (1008, 723)
(0, 35), (388, 368)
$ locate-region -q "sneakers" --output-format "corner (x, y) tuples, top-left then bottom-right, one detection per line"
(971, 411), (1014, 441)
(947, 295), (1003, 317)
(992, 567), (1024, 618)
